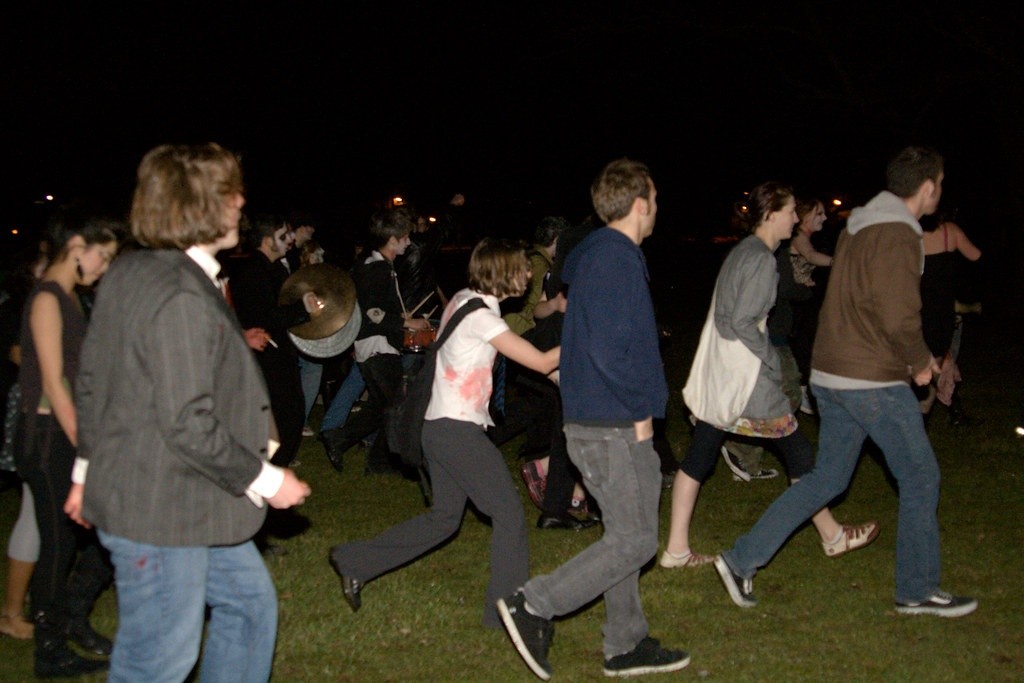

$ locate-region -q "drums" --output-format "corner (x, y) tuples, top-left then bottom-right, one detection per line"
(402, 318), (441, 353)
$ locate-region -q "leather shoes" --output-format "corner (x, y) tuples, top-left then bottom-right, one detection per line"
(536, 506), (598, 529)
(319, 428), (343, 473)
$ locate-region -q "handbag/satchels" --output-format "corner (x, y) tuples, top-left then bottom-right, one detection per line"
(1, 382), (18, 470)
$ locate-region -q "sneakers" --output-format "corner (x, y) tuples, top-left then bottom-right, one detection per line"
(713, 553), (759, 608)
(328, 547), (365, 613)
(721, 445), (752, 482)
(659, 551), (714, 571)
(567, 497), (599, 522)
(821, 520), (880, 558)
(496, 587), (556, 681)
(733, 468), (780, 482)
(893, 584), (979, 617)
(603, 634), (692, 678)
(520, 459), (548, 510)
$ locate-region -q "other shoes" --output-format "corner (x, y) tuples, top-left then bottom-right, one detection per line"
(0, 614), (34, 640)
(288, 459), (302, 467)
(301, 426), (314, 436)
(661, 470), (676, 490)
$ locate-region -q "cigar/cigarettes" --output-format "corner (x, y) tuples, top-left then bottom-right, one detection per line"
(264, 337), (280, 347)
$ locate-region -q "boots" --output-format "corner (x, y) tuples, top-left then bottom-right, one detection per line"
(32, 575), (115, 679)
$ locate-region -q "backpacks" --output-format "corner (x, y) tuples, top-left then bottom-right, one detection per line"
(384, 298), (492, 469)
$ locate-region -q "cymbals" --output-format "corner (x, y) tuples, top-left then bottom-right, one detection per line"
(278, 263), (362, 359)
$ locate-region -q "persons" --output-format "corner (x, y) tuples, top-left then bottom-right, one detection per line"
(328, 233), (596, 628)
(0, 212), (146, 678)
(658, 183), (879, 570)
(912, 204), (991, 417)
(64, 142), (311, 683)
(496, 160), (692, 681)
(713, 148), (979, 618)
(215, 208), (683, 541)
